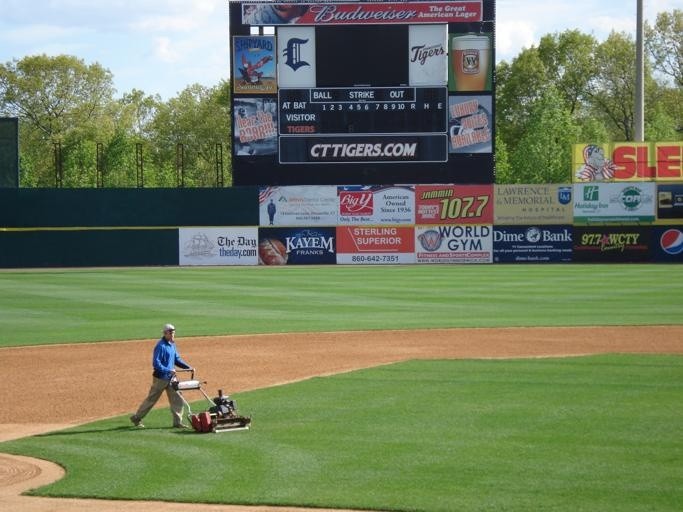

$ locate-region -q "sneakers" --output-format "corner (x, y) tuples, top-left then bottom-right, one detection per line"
(129, 415), (144, 428)
(174, 423), (188, 428)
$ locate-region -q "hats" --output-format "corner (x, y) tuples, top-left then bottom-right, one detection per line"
(162, 324), (176, 333)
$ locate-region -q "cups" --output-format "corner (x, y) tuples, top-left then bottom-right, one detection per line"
(452, 37), (491, 91)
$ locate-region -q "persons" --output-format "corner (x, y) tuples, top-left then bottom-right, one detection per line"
(130, 324), (196, 428)
(267, 199), (277, 225)
